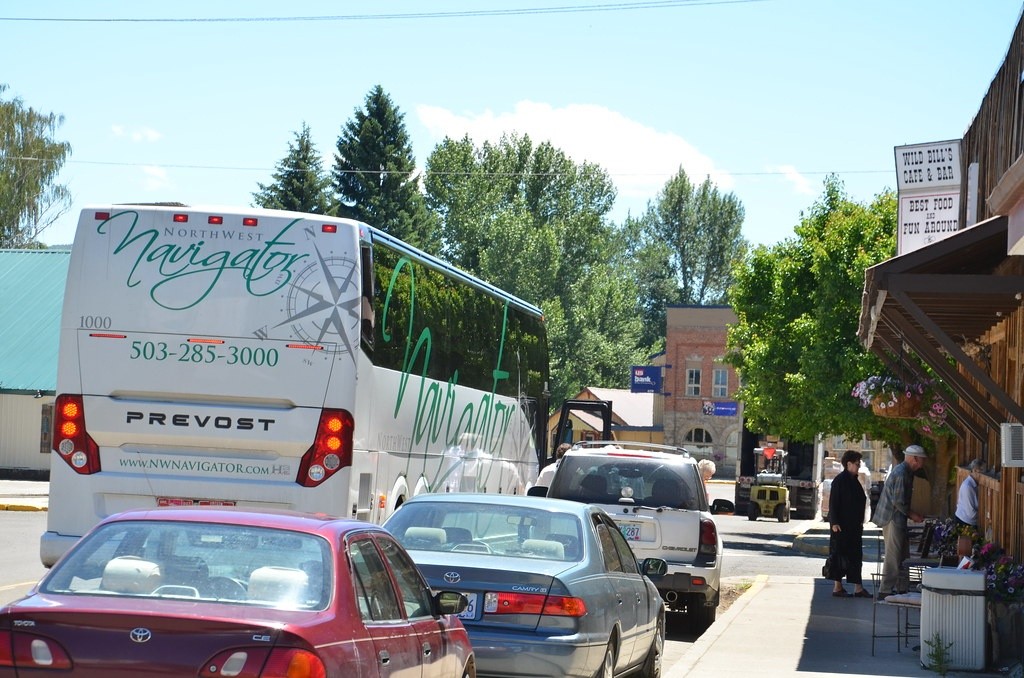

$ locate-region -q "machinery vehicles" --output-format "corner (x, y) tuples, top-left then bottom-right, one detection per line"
(747, 445), (793, 522)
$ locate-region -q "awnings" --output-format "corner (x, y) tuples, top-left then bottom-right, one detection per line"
(856, 216), (1024, 442)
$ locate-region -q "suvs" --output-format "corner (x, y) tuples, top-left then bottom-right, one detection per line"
(545, 439), (725, 636)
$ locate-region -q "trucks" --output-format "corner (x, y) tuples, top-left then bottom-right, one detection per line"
(732, 394), (828, 519)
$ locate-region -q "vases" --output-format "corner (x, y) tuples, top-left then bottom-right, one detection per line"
(871, 389), (920, 419)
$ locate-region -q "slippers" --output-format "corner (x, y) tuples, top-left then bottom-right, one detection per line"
(855, 589), (873, 597)
(833, 588), (852, 597)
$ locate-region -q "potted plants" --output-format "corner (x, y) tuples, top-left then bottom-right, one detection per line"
(985, 556), (1024, 672)
(955, 523), (979, 557)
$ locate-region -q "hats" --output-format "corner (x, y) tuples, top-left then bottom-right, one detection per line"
(903, 445), (929, 459)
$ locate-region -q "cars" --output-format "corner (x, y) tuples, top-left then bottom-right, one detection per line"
(0, 510), (476, 678)
(372, 489), (668, 678)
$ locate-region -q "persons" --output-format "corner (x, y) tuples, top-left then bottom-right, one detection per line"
(955, 458), (987, 529)
(872, 445), (928, 600)
(534, 443), (572, 487)
(821, 450), (876, 598)
(698, 459), (716, 505)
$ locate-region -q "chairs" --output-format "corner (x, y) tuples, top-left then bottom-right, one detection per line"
(870, 572), (921, 658)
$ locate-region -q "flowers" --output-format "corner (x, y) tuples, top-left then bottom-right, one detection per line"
(850, 375), (923, 410)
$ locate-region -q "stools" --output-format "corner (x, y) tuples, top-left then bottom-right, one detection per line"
(903, 557), (940, 593)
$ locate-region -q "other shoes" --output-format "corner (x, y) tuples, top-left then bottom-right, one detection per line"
(878, 592), (894, 600)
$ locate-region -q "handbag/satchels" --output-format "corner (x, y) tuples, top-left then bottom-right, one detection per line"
(823, 533), (850, 582)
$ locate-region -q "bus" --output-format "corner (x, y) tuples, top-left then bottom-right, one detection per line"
(37, 200), (612, 585)
